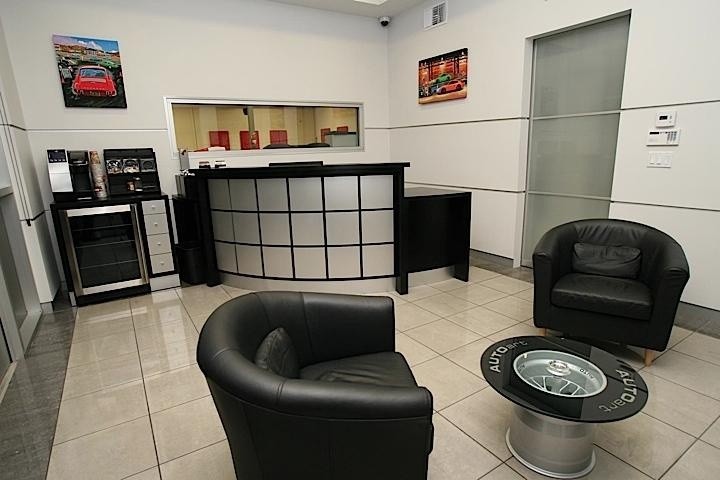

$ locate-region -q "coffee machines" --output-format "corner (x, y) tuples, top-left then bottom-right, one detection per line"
(67, 150), (96, 200)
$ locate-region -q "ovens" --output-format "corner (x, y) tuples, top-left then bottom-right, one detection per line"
(50, 199), (151, 307)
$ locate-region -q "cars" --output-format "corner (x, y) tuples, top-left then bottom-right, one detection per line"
(437, 79), (465, 94)
(432, 74), (451, 84)
(70, 65), (116, 97)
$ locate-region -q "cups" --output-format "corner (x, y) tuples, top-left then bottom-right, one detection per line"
(130, 185), (134, 191)
(89, 150), (108, 198)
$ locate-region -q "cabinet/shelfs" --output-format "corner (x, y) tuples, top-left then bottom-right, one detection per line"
(48, 193), (180, 308)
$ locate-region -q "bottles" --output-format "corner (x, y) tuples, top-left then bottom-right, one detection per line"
(135, 178), (143, 191)
(127, 179), (133, 191)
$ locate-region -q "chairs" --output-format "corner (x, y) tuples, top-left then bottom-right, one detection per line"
(533, 219), (689, 366)
(196, 291), (434, 480)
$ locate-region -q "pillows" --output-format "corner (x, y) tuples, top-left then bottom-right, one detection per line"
(252, 327), (302, 379)
(570, 242), (642, 281)
